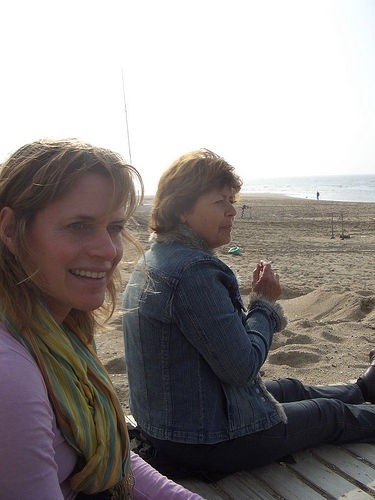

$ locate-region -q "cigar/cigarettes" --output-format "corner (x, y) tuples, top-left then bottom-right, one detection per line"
(260, 261), (273, 267)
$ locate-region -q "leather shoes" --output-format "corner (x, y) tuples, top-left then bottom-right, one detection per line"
(357, 348), (375, 402)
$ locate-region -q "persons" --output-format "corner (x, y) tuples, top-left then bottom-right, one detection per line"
(0, 139), (210, 499)
(317, 191), (320, 199)
(122, 147), (375, 478)
